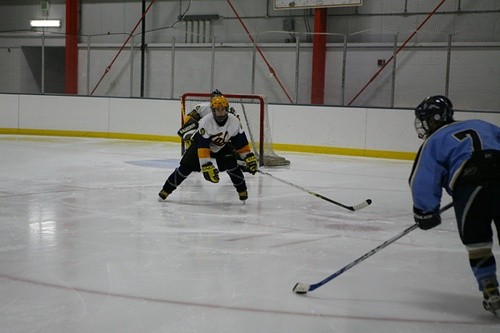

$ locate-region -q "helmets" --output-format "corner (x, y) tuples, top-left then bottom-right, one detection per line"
(210, 95), (229, 122)
(415, 95), (455, 138)
(210, 89), (223, 98)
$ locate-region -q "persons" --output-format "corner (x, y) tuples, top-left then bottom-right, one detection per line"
(159, 89), (258, 200)
(408, 95), (500, 311)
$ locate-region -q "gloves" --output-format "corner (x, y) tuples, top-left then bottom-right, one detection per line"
(412, 203), (441, 230)
(243, 152), (258, 175)
(202, 162), (220, 183)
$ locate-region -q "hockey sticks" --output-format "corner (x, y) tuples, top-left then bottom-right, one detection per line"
(257, 168), (372, 211)
(293, 201), (454, 294)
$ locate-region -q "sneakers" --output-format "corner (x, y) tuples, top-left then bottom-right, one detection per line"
(158, 189), (169, 201)
(483, 282), (500, 321)
(239, 190), (249, 205)
